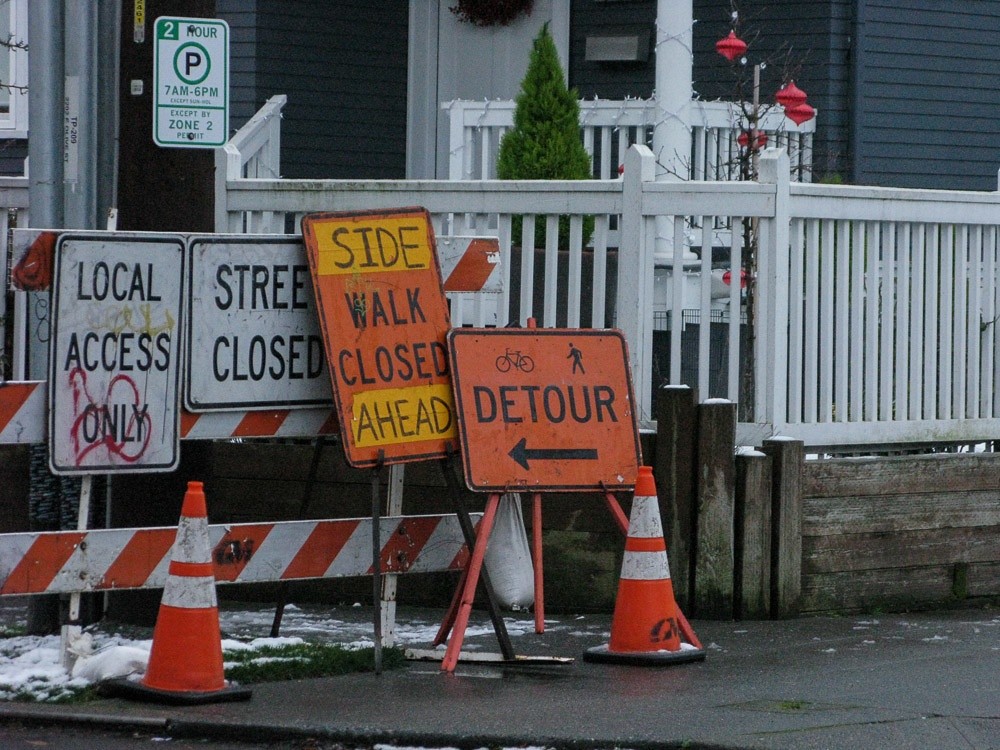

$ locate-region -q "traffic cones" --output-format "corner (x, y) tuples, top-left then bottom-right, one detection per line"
(583, 465), (708, 667)
(105, 482), (253, 707)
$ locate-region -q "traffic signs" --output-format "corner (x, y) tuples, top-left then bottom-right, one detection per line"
(446, 326), (644, 493)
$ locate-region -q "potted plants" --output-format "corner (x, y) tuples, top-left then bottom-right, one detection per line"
(496, 23), (618, 327)
